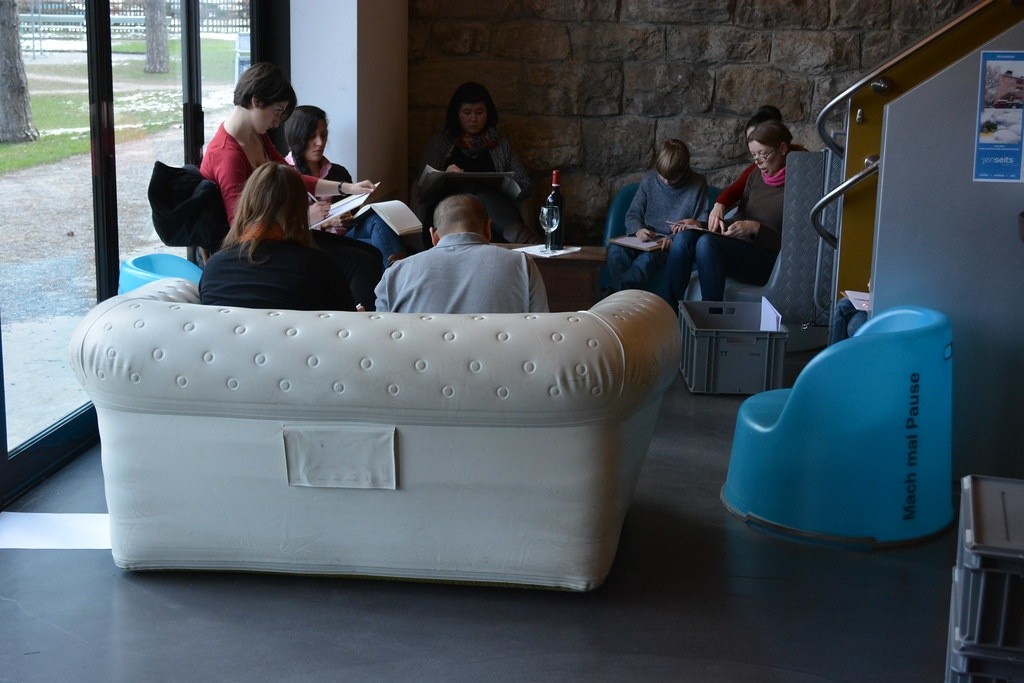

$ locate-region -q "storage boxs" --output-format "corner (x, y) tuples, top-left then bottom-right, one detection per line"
(946, 565), (1024, 683)
(950, 473), (1024, 662)
(670, 293), (788, 397)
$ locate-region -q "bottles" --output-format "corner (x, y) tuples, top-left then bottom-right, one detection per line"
(545, 170), (563, 250)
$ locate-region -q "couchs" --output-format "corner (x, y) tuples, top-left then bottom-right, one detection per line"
(68, 277), (684, 596)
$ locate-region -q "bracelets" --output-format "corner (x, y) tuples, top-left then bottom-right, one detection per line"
(338, 182), (349, 197)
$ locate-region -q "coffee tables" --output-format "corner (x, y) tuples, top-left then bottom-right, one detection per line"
(486, 243), (608, 314)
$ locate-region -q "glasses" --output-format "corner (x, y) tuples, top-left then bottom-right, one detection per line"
(751, 145), (779, 162)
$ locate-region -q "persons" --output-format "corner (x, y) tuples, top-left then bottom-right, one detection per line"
(199, 163), (366, 313)
(374, 195), (551, 315)
(708, 105), (812, 238)
(654, 122), (796, 318)
(286, 104), (408, 283)
(416, 82), (547, 251)
(831, 277), (873, 349)
(597, 138), (711, 308)
(198, 60), (376, 232)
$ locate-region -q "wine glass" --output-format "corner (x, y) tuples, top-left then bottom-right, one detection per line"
(539, 206), (560, 254)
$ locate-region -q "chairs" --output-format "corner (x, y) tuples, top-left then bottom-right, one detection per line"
(119, 253), (205, 292)
(684, 203), (786, 306)
(596, 179), (723, 300)
(717, 305), (958, 548)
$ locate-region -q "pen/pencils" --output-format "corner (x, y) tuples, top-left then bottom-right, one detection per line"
(718, 217), (730, 226)
(307, 191), (319, 203)
